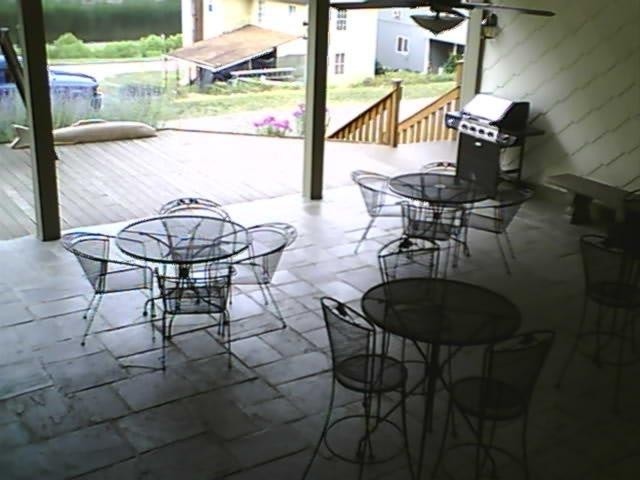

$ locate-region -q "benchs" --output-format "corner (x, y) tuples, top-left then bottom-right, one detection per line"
(545, 173), (629, 224)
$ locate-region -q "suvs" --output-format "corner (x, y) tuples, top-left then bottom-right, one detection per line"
(0, 56), (103, 125)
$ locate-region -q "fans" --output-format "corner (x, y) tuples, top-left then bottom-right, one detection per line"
(328, 0), (556, 36)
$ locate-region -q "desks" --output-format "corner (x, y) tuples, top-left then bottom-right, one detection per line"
(115, 214), (253, 280)
(389, 172), (492, 268)
(361, 276), (523, 431)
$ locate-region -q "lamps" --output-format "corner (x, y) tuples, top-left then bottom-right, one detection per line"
(480, 13), (499, 41)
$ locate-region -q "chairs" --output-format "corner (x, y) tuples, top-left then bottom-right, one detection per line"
(58, 231), (156, 346)
(577, 234), (638, 365)
(154, 268), (240, 374)
(351, 169), (402, 254)
(442, 328), (559, 475)
(393, 199), (466, 267)
(207, 222), (297, 337)
(158, 196), (236, 306)
(304, 296), (408, 460)
(460, 188), (537, 275)
(419, 161), (476, 182)
(378, 237), (441, 280)
